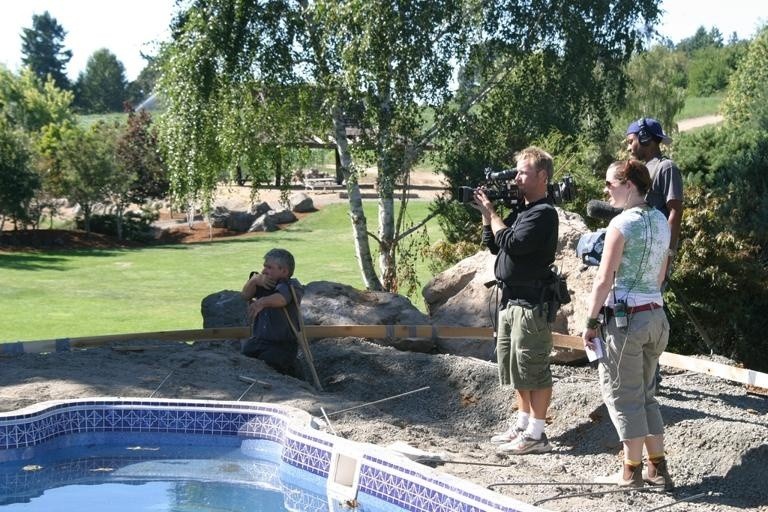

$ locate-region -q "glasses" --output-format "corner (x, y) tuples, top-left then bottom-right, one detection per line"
(605, 179), (629, 188)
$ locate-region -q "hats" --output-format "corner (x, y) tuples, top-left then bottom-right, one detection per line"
(626, 118), (673, 145)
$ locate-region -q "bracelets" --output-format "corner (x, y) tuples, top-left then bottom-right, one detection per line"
(584, 315), (597, 330)
(667, 247), (676, 258)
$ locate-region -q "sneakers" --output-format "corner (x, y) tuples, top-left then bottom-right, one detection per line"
(642, 459), (674, 493)
(491, 425), (524, 444)
(499, 431), (553, 455)
(594, 466), (644, 490)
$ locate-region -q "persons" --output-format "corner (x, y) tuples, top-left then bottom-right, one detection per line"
(581, 158), (676, 493)
(241, 246), (309, 383)
(624, 117), (684, 395)
(468, 145), (560, 456)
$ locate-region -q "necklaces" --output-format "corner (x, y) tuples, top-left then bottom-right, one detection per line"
(629, 201), (649, 208)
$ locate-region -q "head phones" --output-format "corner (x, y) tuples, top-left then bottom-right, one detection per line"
(638, 116), (651, 147)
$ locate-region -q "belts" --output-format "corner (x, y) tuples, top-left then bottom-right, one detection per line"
(605, 301), (661, 315)
